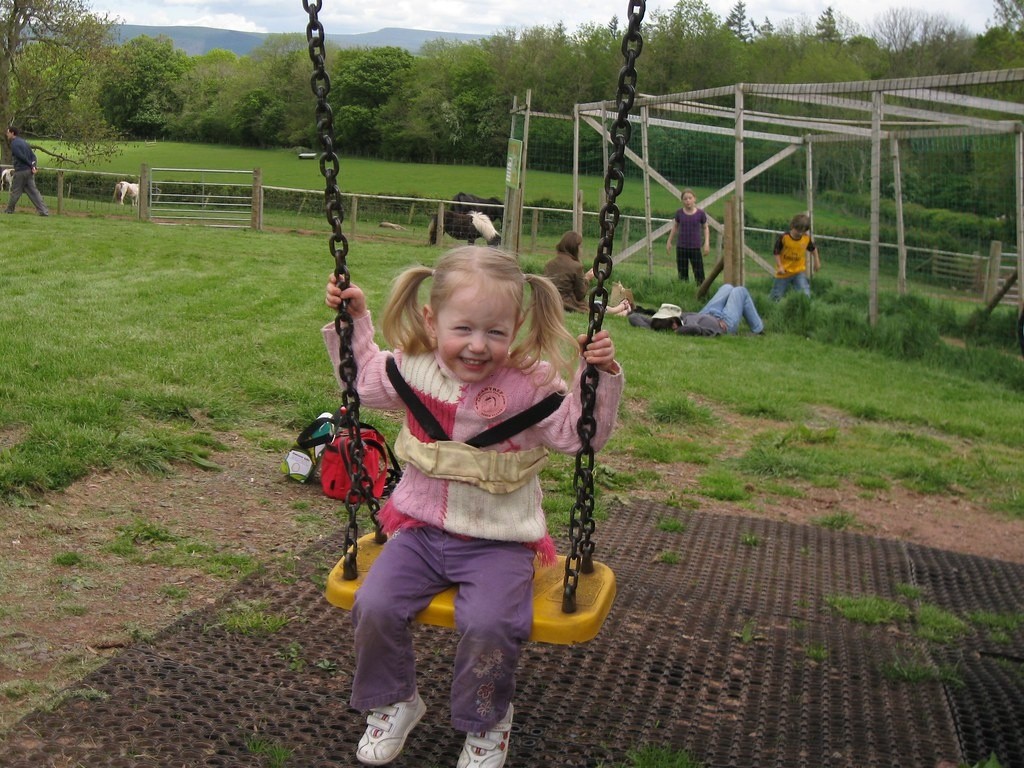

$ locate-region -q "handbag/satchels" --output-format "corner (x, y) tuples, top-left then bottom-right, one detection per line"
(609, 281), (636, 312)
(296, 417), (400, 503)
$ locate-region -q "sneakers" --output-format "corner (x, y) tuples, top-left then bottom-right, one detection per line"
(355, 684), (427, 765)
(455, 701), (514, 768)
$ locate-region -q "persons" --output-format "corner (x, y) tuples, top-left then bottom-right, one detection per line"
(666, 190), (710, 287)
(543, 231), (634, 317)
(4, 127), (48, 216)
(321, 244), (623, 768)
(770, 214), (821, 304)
(629, 283), (766, 337)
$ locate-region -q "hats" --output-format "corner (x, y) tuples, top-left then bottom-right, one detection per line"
(652, 304), (683, 319)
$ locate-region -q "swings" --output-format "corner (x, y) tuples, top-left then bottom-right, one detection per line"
(299, 0), (650, 647)
